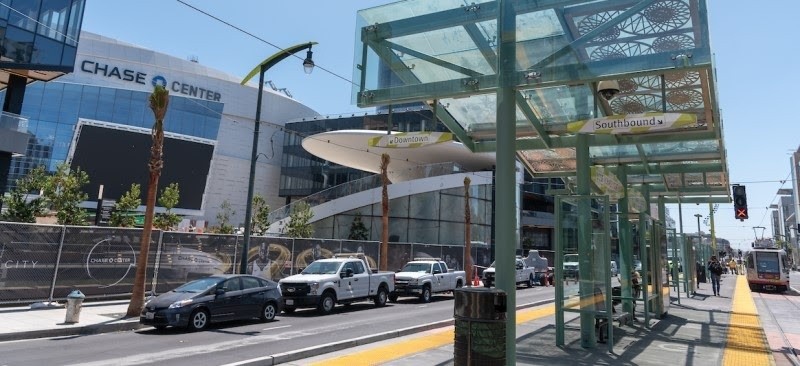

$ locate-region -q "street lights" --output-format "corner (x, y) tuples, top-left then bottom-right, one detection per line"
(239, 40), (319, 274)
(694, 214), (703, 266)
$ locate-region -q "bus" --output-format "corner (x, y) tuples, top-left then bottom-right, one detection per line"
(744, 226), (791, 294)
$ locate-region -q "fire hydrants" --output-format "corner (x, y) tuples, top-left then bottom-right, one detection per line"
(64, 289), (86, 323)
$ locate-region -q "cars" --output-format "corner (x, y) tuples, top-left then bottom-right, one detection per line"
(139, 273), (283, 334)
(563, 253), (579, 281)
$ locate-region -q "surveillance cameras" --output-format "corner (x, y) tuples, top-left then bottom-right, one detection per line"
(597, 80), (620, 100)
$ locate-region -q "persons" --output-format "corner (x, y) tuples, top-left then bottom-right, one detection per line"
(708, 255), (721, 296)
(728, 259), (737, 274)
(618, 267), (640, 315)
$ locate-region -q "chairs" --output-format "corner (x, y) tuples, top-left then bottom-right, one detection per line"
(593, 289), (629, 343)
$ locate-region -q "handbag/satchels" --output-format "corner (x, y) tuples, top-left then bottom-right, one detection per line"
(708, 262), (723, 276)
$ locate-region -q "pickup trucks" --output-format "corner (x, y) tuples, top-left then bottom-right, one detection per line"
(277, 257), (395, 315)
(482, 256), (536, 289)
(387, 257), (466, 303)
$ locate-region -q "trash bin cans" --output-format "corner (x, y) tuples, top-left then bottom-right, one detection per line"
(453, 285), (507, 366)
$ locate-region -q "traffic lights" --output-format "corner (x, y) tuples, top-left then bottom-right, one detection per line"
(732, 185), (749, 220)
